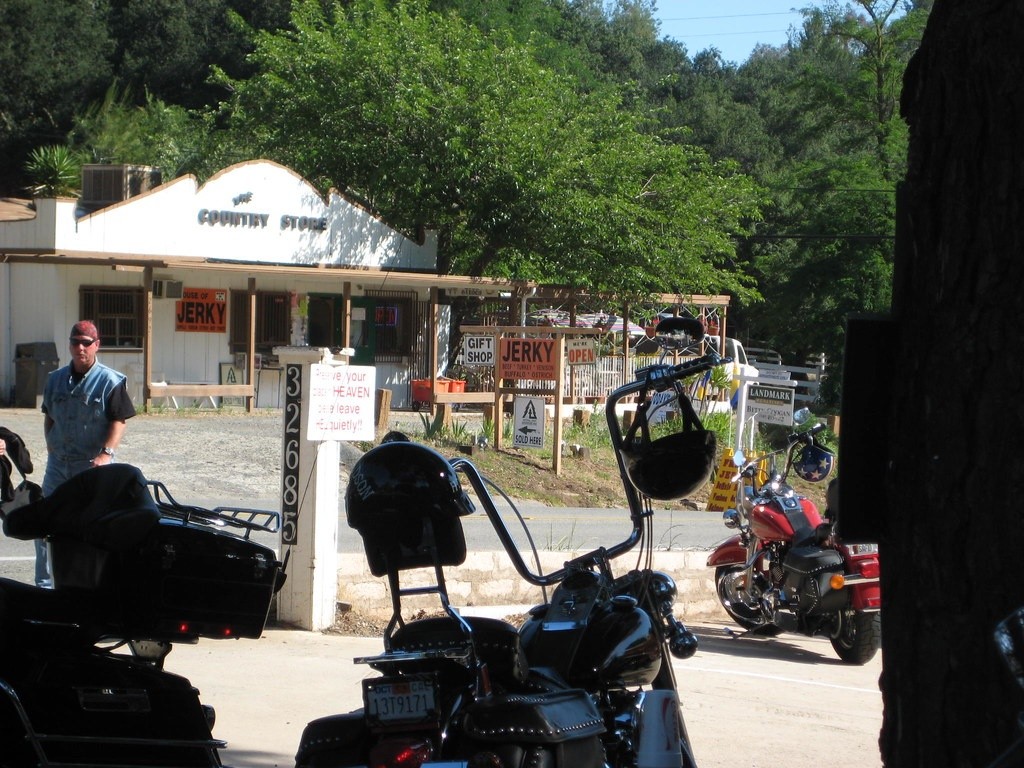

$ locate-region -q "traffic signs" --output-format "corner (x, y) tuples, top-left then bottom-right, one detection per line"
(513, 395), (546, 448)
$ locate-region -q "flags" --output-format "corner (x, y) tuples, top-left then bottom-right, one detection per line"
(698, 370), (712, 400)
(729, 364), (740, 410)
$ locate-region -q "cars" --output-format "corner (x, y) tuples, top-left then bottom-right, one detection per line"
(688, 339), (793, 403)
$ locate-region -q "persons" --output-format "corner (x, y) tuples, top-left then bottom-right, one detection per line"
(0, 438), (6, 456)
(34, 320), (137, 589)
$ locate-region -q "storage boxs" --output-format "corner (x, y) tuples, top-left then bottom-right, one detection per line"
(448, 380), (466, 393)
(412, 380), (450, 402)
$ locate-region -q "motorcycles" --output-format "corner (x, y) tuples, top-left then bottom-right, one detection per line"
(705, 405), (881, 666)
(294, 313), (731, 767)
(0, 482), (291, 768)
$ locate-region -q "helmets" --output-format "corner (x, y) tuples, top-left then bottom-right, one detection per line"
(346, 442), (476, 528)
(791, 443), (834, 483)
(615, 428), (717, 501)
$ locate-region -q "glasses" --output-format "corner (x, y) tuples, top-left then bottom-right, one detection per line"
(70, 337), (98, 347)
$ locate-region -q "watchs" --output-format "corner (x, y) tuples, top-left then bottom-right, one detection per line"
(99, 447), (115, 461)
